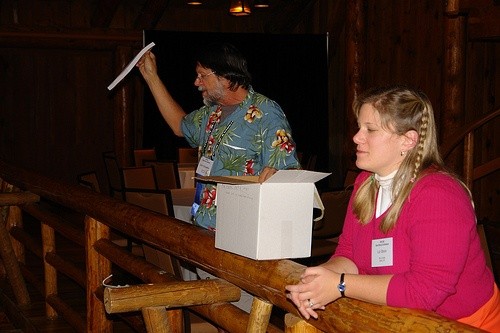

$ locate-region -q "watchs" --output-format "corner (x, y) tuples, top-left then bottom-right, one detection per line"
(339, 271), (349, 300)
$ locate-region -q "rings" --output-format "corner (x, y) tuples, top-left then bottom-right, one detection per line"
(308, 299), (314, 307)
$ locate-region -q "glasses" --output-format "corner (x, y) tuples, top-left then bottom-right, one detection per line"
(195, 69), (217, 82)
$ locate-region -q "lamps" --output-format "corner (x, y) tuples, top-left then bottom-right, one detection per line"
(227, 0), (251, 16)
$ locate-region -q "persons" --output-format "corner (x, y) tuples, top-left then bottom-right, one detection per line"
(135, 44), (303, 314)
(284, 84), (500, 333)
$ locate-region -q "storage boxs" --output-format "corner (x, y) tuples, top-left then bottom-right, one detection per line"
(192, 169), (332, 260)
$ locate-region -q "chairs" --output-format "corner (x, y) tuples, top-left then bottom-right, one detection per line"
(78, 146), (362, 333)
